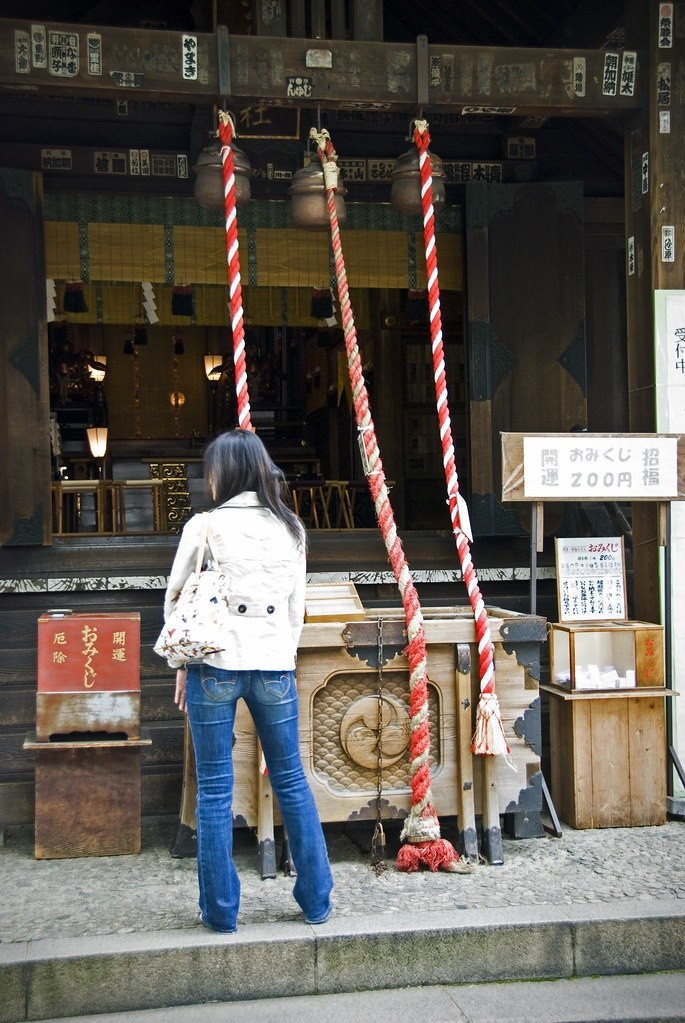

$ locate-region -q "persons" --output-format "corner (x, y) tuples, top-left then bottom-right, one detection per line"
(163, 431), (335, 934)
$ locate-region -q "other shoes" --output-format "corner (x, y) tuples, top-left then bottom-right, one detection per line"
(200, 912), (203, 924)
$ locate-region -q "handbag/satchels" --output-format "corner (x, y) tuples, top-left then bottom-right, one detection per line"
(153, 512), (230, 662)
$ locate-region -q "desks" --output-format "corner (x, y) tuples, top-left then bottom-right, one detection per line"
(539, 685), (681, 829)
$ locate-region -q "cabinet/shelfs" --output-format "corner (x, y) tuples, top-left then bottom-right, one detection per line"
(400, 335), (472, 530)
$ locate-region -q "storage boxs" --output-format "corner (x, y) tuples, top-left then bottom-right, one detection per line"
(548, 620), (667, 695)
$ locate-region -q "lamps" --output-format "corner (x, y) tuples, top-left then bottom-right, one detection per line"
(202, 348), (224, 438)
(86, 354), (108, 426)
(82, 418), (109, 480)
